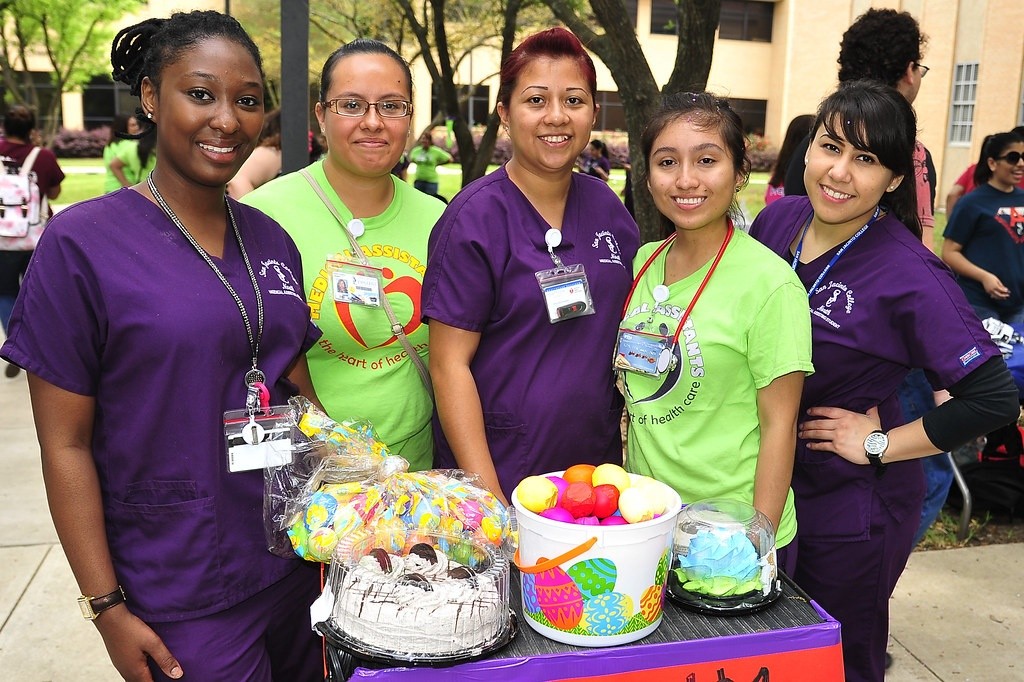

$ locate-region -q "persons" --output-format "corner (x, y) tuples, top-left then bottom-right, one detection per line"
(0, 7), (326, 682)
(765, 115), (815, 204)
(1, 105), (65, 378)
(578, 140), (610, 181)
(243, 38), (447, 475)
(838, 8), (936, 251)
(228, 83), (322, 200)
(337, 280), (347, 293)
(421, 30), (639, 508)
(942, 126), (1024, 418)
(614, 91), (814, 578)
(748, 83), (1019, 682)
(621, 155), (635, 219)
(103, 113), (156, 194)
(392, 133), (453, 195)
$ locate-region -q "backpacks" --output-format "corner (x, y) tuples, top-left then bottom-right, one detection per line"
(0, 147), (49, 251)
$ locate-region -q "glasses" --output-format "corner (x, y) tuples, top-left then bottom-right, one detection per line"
(915, 62), (930, 78)
(320, 98), (412, 118)
(994, 151), (1024, 165)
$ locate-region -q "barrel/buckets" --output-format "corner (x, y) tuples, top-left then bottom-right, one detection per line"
(507, 467), (682, 646)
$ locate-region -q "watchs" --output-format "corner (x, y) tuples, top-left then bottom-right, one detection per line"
(863, 430), (889, 467)
(78, 586), (126, 620)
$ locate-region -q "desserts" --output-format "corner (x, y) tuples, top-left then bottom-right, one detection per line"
(672, 522), (762, 595)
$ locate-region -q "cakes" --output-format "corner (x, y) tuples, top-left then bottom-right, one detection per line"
(331, 543), (503, 655)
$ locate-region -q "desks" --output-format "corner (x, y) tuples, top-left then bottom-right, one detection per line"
(326, 564), (845, 682)
